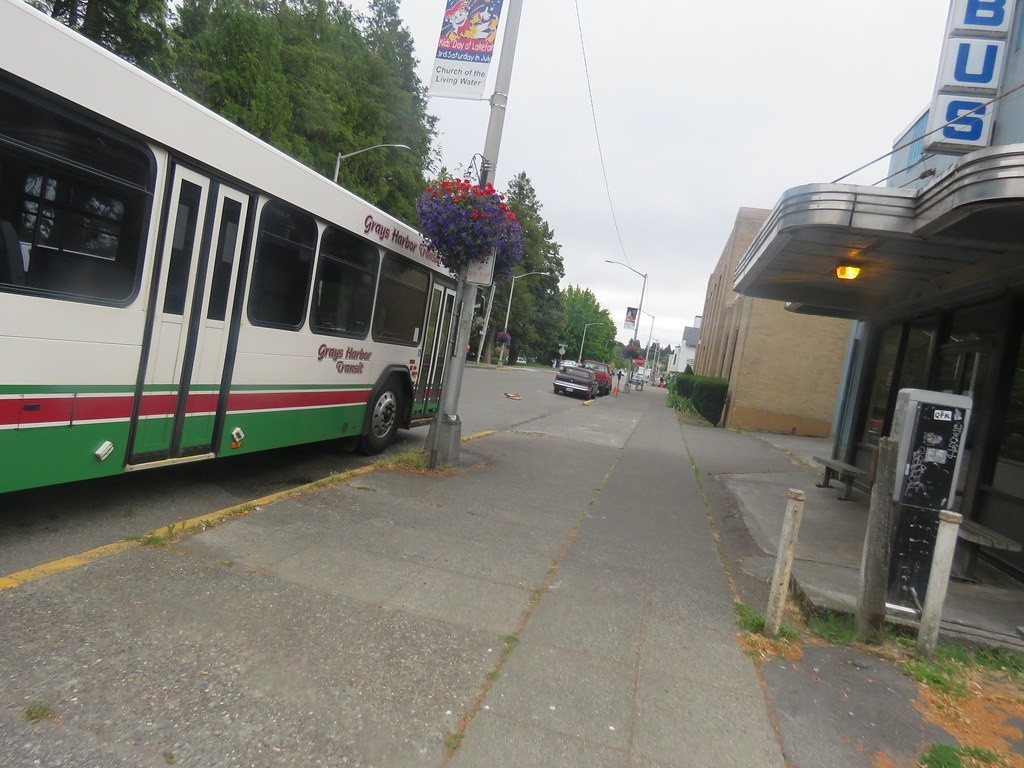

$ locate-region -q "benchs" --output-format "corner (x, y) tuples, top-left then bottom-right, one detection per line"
(950, 517), (1021, 585)
(811, 443), (875, 502)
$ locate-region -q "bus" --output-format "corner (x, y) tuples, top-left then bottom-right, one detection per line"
(0, 0), (484, 498)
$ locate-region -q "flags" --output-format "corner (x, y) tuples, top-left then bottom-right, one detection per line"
(623, 306), (638, 329)
(425, 0), (504, 101)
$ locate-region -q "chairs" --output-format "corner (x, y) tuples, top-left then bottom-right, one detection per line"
(0, 221), (26, 285)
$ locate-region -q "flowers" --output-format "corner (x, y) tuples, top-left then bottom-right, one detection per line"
(496, 332), (511, 347)
(415, 179), (525, 281)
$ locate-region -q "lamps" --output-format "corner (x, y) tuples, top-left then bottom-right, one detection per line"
(836, 265), (861, 280)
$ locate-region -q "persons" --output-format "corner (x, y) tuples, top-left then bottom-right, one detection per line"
(551, 358), (557, 370)
(465, 344), (470, 362)
(659, 375), (668, 390)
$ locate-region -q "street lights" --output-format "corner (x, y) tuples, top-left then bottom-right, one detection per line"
(496, 271), (551, 365)
(645, 347), (661, 373)
(333, 143), (410, 184)
(578, 323), (604, 364)
(604, 259), (648, 382)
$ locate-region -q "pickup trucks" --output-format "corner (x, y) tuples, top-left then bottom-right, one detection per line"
(579, 359), (616, 397)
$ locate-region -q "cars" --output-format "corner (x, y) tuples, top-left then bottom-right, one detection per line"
(559, 360), (576, 373)
(552, 365), (599, 400)
(629, 374), (642, 385)
